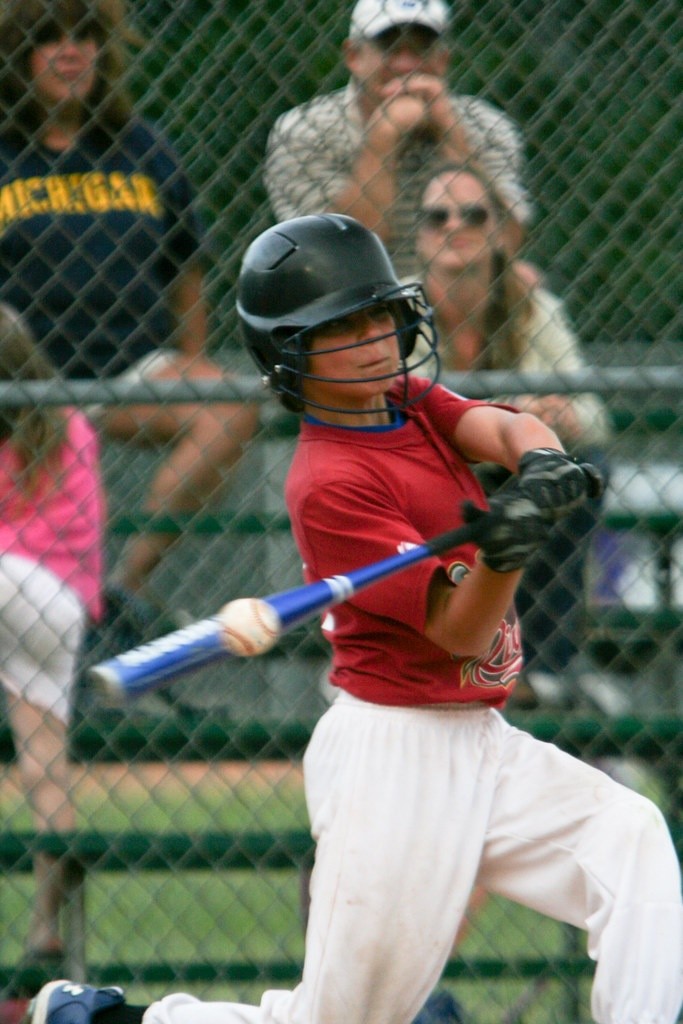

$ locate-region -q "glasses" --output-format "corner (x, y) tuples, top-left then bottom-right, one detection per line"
(418, 204), (488, 229)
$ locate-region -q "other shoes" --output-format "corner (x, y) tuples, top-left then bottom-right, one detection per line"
(509, 671), (540, 709)
(104, 574), (165, 635)
(0, 948), (66, 1000)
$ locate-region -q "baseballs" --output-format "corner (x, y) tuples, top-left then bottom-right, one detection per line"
(217, 596), (282, 659)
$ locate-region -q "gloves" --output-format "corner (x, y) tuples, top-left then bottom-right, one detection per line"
(519, 447), (590, 522)
(462, 496), (551, 572)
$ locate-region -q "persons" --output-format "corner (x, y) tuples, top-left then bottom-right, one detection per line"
(0, 303), (104, 994)
(22, 214), (683, 1024)
(264, 0), (532, 656)
(0, 0), (259, 643)
(398, 163), (606, 710)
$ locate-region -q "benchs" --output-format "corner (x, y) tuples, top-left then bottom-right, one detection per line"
(0, 351), (683, 1024)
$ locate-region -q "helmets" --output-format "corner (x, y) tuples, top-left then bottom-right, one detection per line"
(234, 211), (440, 413)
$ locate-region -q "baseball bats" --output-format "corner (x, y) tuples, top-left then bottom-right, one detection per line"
(85, 517), (495, 713)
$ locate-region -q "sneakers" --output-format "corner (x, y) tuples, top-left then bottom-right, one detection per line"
(27, 980), (124, 1024)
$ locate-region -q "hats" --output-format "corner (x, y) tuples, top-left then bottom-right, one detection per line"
(348, 0), (448, 40)
